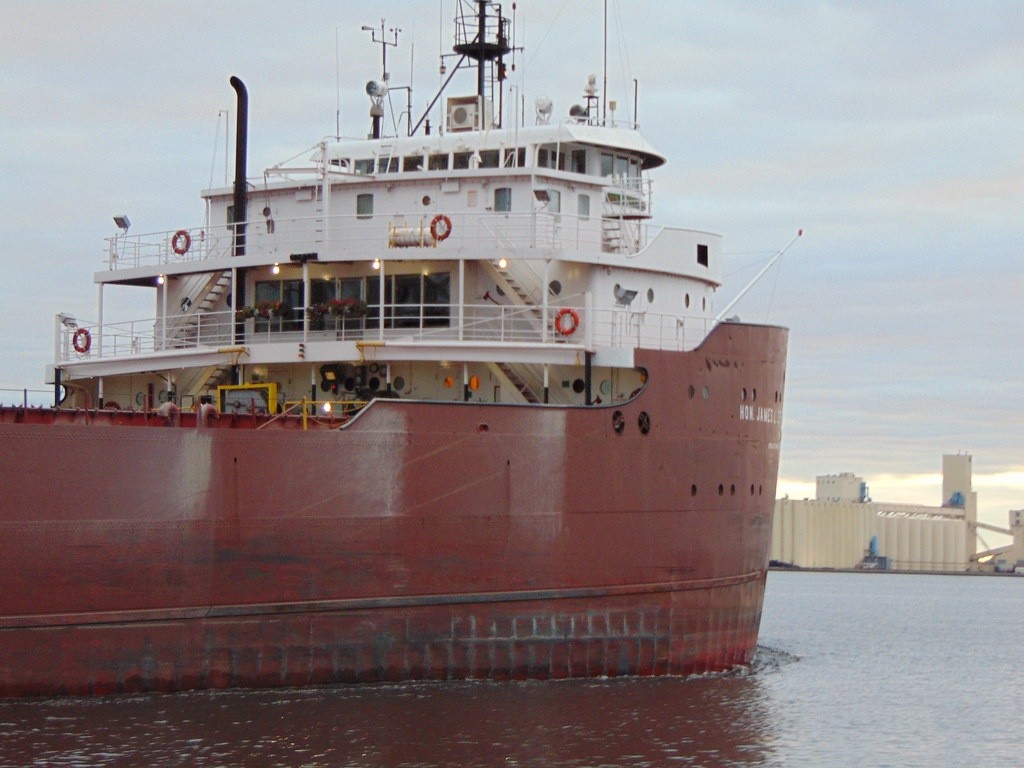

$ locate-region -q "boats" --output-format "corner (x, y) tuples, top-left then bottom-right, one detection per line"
(3, 1), (789, 693)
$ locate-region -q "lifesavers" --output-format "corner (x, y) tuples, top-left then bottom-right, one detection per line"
(554, 309), (579, 335)
(430, 214), (452, 240)
(71, 329), (93, 352)
(171, 230), (191, 254)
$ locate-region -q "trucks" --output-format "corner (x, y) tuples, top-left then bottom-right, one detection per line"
(1013, 561), (1023, 576)
(862, 556), (893, 569)
(992, 559), (1014, 572)
